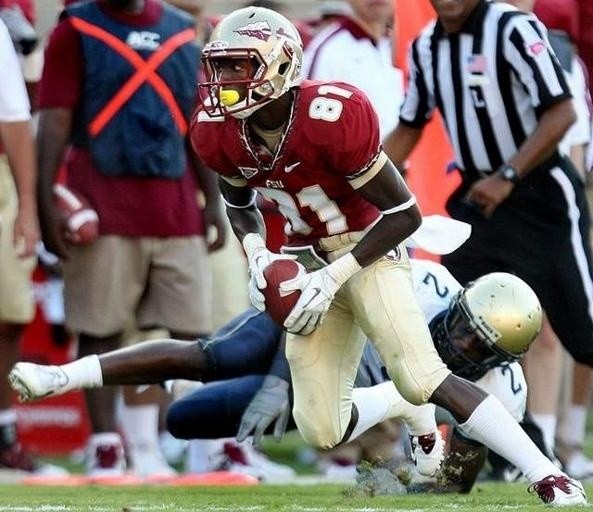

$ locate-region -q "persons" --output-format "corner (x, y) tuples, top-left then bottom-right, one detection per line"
(1, 2), (590, 505)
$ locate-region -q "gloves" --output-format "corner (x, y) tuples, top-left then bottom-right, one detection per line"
(352, 466), (409, 499)
(280, 251), (364, 337)
(242, 231), (299, 314)
(233, 373), (290, 448)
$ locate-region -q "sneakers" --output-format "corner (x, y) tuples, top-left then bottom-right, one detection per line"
(405, 401), (453, 479)
(529, 468), (588, 507)
(188, 440), (296, 485)
(0, 442), (70, 481)
(6, 362), (70, 404)
(87, 434), (127, 481)
(326, 452), (355, 482)
(126, 436), (179, 480)
(486, 433), (593, 484)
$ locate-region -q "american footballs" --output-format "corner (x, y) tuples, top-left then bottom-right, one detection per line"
(260, 260), (318, 336)
(50, 185), (100, 245)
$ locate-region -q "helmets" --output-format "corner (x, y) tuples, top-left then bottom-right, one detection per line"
(429, 270), (544, 381)
(196, 5), (304, 120)
